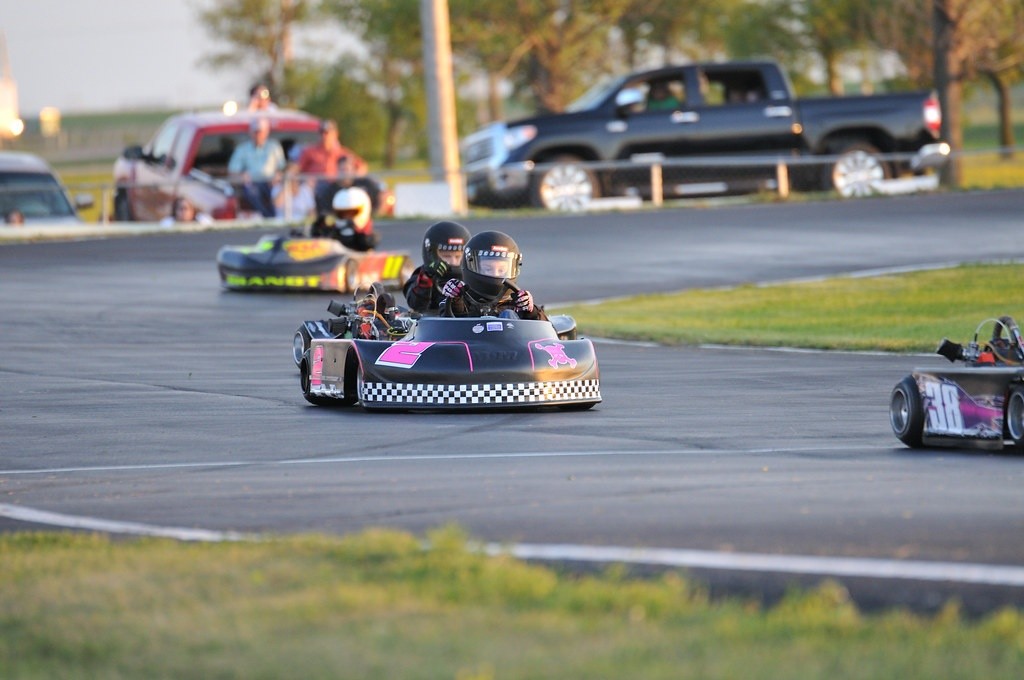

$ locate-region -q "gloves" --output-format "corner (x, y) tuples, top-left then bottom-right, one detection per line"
(441, 278), (470, 299)
(424, 258), (452, 279)
(513, 290), (534, 313)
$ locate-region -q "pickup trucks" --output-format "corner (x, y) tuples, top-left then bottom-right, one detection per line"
(111, 107), (396, 222)
(460, 59), (952, 214)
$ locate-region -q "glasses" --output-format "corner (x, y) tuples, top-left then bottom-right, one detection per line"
(334, 207), (362, 220)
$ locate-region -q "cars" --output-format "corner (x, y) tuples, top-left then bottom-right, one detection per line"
(0, 149), (94, 233)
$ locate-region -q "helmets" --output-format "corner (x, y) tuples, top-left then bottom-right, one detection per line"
(331, 186), (372, 229)
(459, 229), (523, 300)
(421, 221), (472, 285)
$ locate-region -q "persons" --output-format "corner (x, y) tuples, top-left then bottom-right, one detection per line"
(645, 79), (680, 112)
(5, 207), (23, 226)
(248, 81), (279, 113)
(441, 230), (559, 337)
(327, 185), (381, 252)
(228, 117), (286, 219)
(160, 195), (215, 225)
(286, 117), (381, 216)
(403, 220), (472, 312)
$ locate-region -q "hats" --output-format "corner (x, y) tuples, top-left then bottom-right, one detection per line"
(250, 117), (267, 131)
